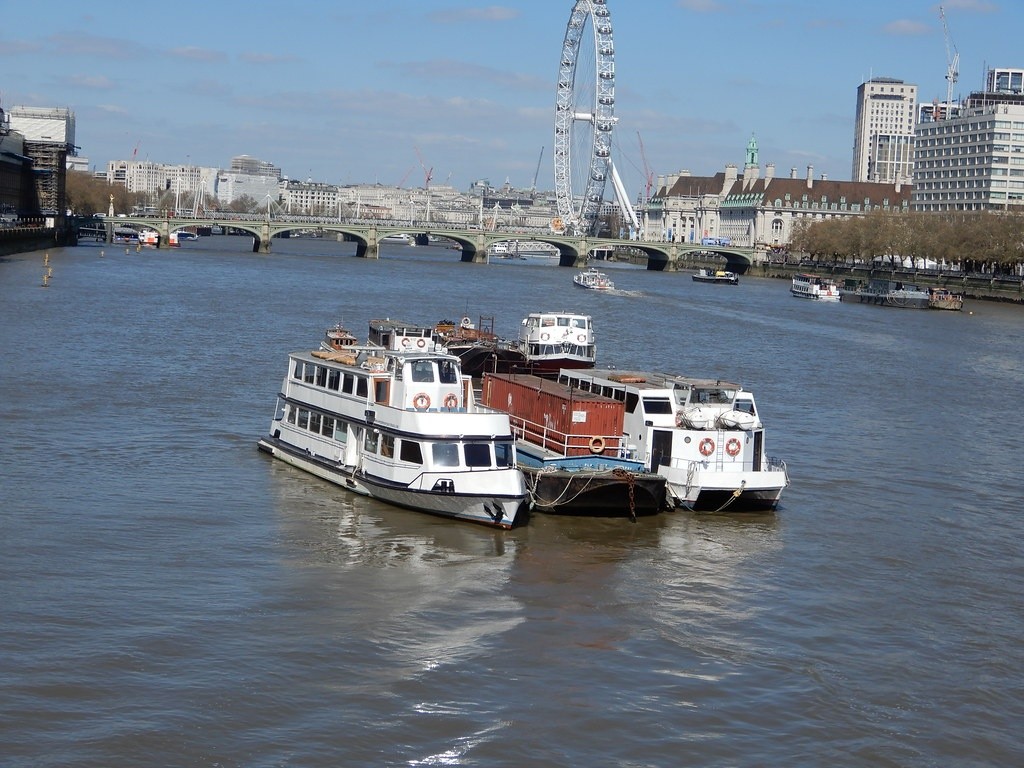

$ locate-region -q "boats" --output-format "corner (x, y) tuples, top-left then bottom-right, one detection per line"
(139, 227), (180, 247)
(692, 266), (741, 285)
(572, 267), (616, 293)
(256, 314), (528, 529)
(838, 272), (933, 309)
(789, 272), (838, 302)
(555, 366), (789, 515)
(114, 232), (138, 244)
(507, 311), (597, 371)
(421, 319), (670, 517)
(174, 231), (198, 241)
(928, 285), (964, 312)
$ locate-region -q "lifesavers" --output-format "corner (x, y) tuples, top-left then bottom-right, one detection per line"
(699, 438), (715, 456)
(413, 393), (430, 411)
(402, 338), (410, 347)
(577, 334), (586, 343)
(462, 317), (471, 326)
(726, 439), (741, 456)
(417, 339), (425, 348)
(540, 332), (550, 341)
(443, 393), (458, 412)
(588, 435), (606, 453)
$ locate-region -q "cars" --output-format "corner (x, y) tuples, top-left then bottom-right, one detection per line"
(93, 212), (106, 217)
